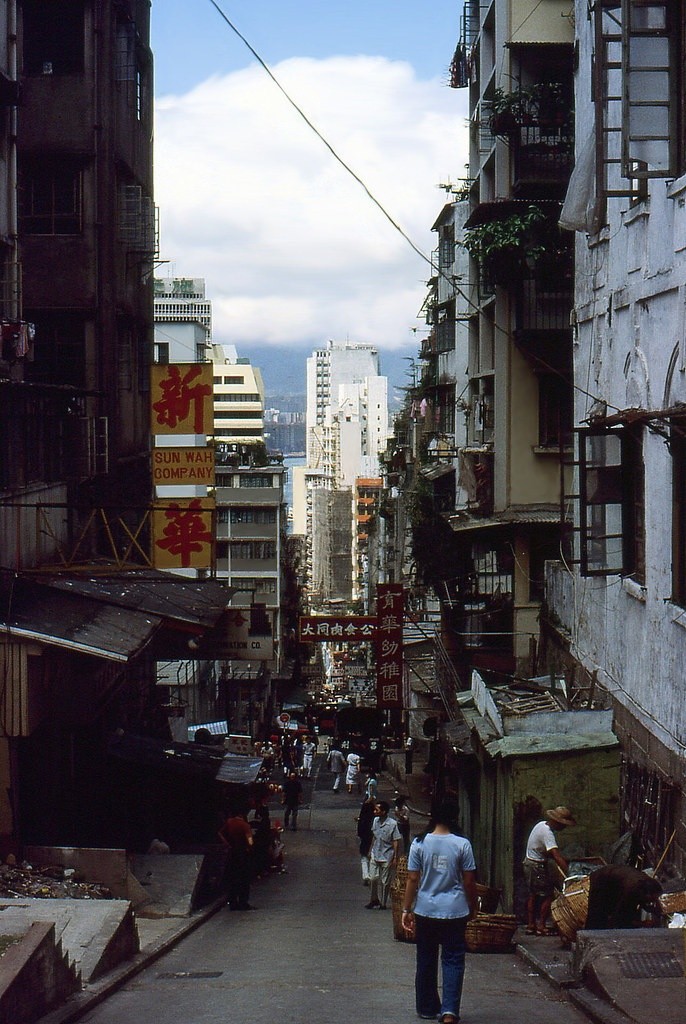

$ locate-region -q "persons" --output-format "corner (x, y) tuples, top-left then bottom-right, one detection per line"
(346, 749), (360, 794)
(281, 772), (299, 831)
(326, 744), (347, 791)
(218, 801), (258, 910)
(357, 769), (411, 911)
(260, 734), (319, 778)
(523, 806), (574, 936)
(402, 792), (478, 1024)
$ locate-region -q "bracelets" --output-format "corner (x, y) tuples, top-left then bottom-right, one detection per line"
(402, 910), (411, 913)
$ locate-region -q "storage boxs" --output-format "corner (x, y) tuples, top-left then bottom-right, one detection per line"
(555, 855), (608, 893)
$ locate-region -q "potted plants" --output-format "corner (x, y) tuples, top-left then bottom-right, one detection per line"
(483, 82), (571, 130)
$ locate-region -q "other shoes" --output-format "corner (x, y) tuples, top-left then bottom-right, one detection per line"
(443, 1014), (455, 1024)
(230, 902), (257, 911)
(333, 788), (339, 793)
(348, 789), (352, 793)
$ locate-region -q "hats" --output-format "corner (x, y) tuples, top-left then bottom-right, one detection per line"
(547, 806), (578, 826)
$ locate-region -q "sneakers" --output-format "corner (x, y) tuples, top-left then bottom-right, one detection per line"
(365, 899), (386, 910)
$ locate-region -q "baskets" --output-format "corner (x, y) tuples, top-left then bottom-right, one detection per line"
(463, 910), (520, 954)
(659, 890), (686, 915)
(550, 875), (591, 940)
(391, 856), (420, 944)
